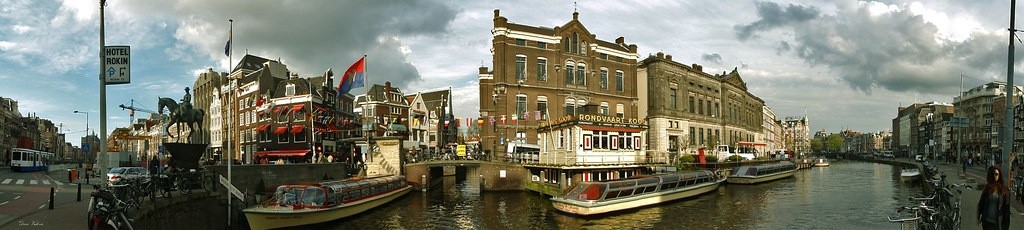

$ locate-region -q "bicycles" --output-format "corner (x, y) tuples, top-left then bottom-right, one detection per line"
(88, 166), (215, 230)
(888, 161), (973, 230)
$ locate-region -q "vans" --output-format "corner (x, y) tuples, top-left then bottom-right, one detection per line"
(507, 143), (540, 163)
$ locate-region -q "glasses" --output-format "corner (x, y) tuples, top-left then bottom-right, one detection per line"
(991, 173), (1000, 176)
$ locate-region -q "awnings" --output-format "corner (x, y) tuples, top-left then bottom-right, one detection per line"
(739, 141), (766, 146)
(389, 124), (406, 131)
(253, 150), (311, 157)
(254, 105), (305, 135)
(578, 124), (640, 133)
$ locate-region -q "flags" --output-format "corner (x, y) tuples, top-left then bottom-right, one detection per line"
(309, 107), (389, 130)
(392, 111), (543, 127)
(235, 88), (308, 121)
(225, 27), (232, 56)
(336, 57), (364, 100)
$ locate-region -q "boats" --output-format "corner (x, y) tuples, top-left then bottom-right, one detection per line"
(727, 161), (796, 184)
(241, 174), (413, 230)
(901, 168), (921, 184)
(814, 156), (830, 167)
(549, 169), (727, 216)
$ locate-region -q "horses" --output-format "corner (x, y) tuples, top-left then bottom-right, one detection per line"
(158, 95), (205, 144)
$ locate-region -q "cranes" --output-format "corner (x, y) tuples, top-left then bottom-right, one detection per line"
(119, 99), (156, 125)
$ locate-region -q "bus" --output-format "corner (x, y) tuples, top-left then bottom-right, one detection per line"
(11, 148), (55, 173)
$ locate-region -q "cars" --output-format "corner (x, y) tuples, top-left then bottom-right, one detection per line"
(106, 167), (167, 187)
(466, 145), (476, 155)
(916, 156), (926, 162)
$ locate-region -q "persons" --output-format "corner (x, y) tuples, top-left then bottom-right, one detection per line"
(311, 152), (334, 164)
(404, 142), (483, 163)
(251, 155), (291, 165)
(150, 156), (159, 176)
(179, 87), (191, 116)
(978, 166), (1011, 230)
(938, 154), (990, 172)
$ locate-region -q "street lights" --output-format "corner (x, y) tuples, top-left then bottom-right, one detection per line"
(74, 111), (88, 164)
